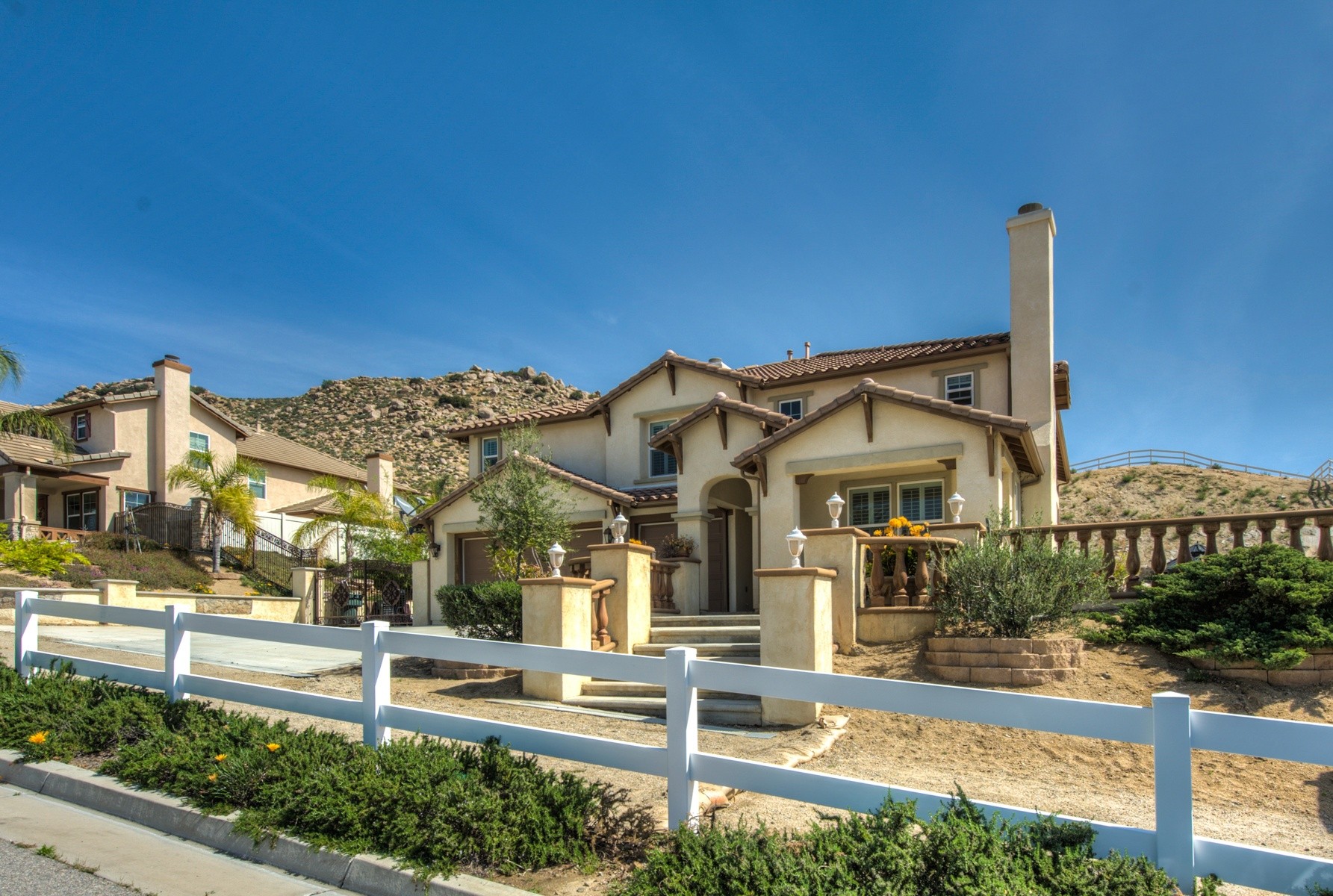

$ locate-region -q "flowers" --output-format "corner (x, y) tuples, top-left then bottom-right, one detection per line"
(864, 516), (934, 574)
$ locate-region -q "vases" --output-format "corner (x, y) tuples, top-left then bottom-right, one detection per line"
(866, 575), (914, 606)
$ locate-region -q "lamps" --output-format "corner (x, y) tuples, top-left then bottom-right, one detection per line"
(603, 525), (612, 544)
(547, 539), (565, 576)
(785, 526), (808, 568)
(947, 492), (966, 522)
(428, 541), (441, 557)
(825, 491), (845, 527)
(611, 511), (629, 543)
